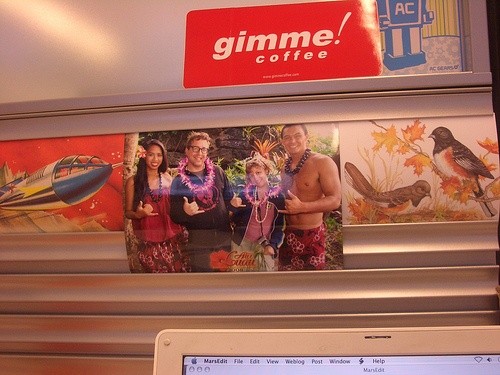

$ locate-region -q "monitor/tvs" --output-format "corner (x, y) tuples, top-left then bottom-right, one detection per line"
(152, 326), (500, 375)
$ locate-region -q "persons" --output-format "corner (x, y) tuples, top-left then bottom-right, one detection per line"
(277, 124), (341, 271)
(169, 130), (239, 272)
(226, 157), (286, 272)
(125, 140), (189, 273)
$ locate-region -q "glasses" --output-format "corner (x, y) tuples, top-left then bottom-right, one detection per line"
(188, 146), (209, 154)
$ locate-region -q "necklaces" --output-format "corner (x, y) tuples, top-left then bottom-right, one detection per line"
(255, 185), (269, 223)
(177, 156), (215, 205)
(144, 171), (163, 203)
(284, 148), (312, 177)
(243, 181), (282, 204)
